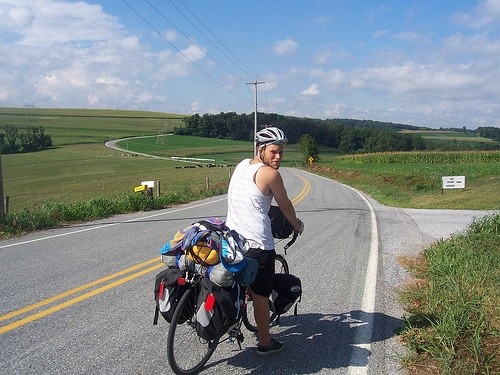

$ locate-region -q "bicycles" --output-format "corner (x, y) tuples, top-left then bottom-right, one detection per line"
(168, 218), (300, 375)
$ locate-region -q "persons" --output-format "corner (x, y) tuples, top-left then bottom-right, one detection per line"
(226, 127), (304, 355)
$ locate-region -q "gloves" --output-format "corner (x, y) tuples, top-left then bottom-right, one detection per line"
(297, 218), (304, 233)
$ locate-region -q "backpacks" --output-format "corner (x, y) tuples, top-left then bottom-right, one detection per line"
(153, 265), (246, 340)
(271, 273), (303, 325)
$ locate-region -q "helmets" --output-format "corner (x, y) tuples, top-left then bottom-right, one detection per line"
(254, 126), (288, 147)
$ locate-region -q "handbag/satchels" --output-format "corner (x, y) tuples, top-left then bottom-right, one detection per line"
(267, 203), (294, 238)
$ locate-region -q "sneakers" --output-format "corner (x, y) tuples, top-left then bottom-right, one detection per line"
(256, 337), (284, 354)
